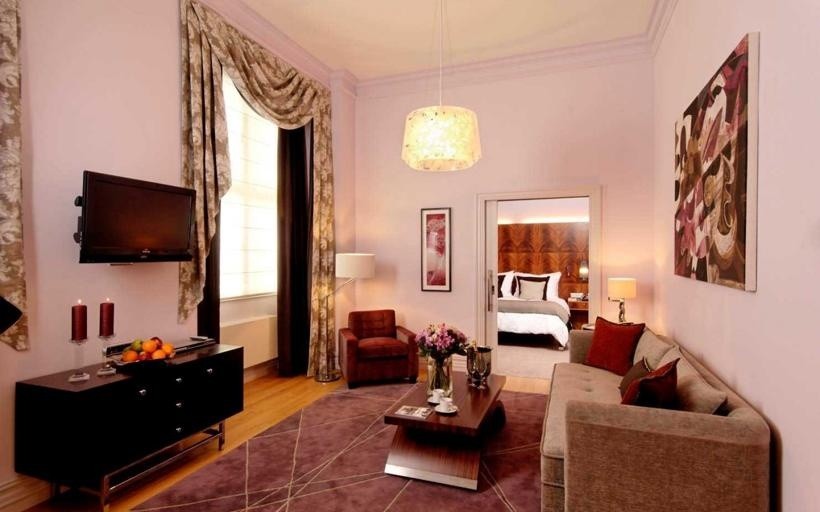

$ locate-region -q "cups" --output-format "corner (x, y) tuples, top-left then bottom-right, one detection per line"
(433, 388), (446, 401)
(439, 398), (453, 412)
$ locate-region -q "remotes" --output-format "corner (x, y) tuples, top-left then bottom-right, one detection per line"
(190, 335), (208, 341)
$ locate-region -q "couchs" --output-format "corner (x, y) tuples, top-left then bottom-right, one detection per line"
(337, 306), (419, 390)
(536, 322), (774, 512)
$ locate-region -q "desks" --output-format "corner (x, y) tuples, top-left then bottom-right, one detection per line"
(580, 322), (601, 332)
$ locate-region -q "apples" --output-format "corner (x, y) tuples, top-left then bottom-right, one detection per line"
(132, 339), (143, 352)
(138, 352), (149, 361)
(150, 336), (162, 349)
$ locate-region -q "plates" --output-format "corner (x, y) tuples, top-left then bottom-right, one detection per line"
(427, 396), (451, 404)
(435, 404), (458, 413)
(113, 355), (168, 368)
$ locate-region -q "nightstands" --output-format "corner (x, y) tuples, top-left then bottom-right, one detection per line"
(565, 297), (589, 330)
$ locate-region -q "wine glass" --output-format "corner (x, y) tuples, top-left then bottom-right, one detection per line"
(476, 360), (487, 389)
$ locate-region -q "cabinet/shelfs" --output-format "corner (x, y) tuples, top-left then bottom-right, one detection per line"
(10, 336), (244, 510)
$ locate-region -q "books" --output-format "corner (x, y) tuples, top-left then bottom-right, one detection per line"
(394, 405), (433, 419)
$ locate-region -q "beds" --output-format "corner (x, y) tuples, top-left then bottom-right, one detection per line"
(495, 294), (572, 354)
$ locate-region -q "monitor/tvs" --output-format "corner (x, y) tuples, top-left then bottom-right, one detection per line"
(74, 170), (196, 264)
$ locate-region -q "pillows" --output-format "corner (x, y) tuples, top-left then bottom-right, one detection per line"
(581, 315), (646, 376)
(617, 356), (652, 400)
(633, 326), (676, 372)
(619, 358), (681, 410)
(497, 269), (562, 301)
(654, 344), (728, 416)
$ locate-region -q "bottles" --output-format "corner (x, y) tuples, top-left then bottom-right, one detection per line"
(472, 341), (478, 352)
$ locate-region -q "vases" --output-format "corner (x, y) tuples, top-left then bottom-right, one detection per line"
(423, 354), (453, 396)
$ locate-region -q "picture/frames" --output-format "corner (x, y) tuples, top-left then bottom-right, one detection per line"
(418, 206), (453, 293)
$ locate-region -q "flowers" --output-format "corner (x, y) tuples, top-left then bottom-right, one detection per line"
(411, 321), (470, 391)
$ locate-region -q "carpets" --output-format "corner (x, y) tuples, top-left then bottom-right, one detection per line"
(124, 379), (551, 511)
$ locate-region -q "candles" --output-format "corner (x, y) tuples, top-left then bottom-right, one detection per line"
(97, 298), (115, 339)
(68, 298), (88, 343)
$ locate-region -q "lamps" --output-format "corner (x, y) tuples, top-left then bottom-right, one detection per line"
(312, 250), (378, 383)
(575, 258), (589, 301)
(400, 0), (484, 173)
(606, 276), (637, 325)
